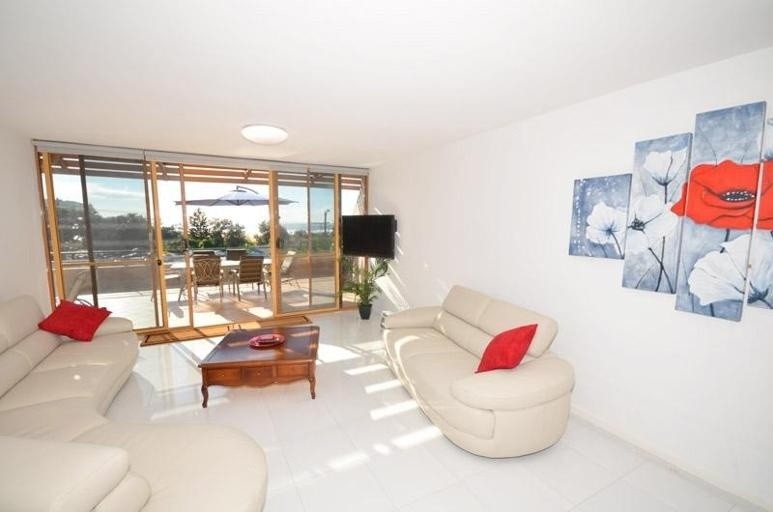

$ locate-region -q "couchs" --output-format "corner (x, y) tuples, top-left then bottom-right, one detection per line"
(0, 296), (151, 511)
(377, 283), (581, 454)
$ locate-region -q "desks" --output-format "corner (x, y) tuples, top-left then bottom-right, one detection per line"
(168, 258), (271, 296)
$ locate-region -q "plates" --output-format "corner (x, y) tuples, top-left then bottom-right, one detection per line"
(248, 333), (285, 348)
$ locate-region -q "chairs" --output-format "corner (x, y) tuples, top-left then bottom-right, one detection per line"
(192, 255), (221, 303)
(276, 250), (302, 293)
(192, 251), (214, 255)
(226, 249), (247, 292)
(233, 255), (267, 303)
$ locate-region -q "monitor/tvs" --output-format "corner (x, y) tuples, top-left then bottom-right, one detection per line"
(341, 214), (396, 260)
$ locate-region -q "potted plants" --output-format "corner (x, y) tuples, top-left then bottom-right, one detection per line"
(341, 254), (391, 320)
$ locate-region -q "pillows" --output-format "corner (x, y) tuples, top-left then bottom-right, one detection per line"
(37, 301), (110, 341)
(478, 323), (542, 373)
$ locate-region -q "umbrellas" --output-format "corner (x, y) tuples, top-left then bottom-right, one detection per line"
(174, 185), (299, 206)
